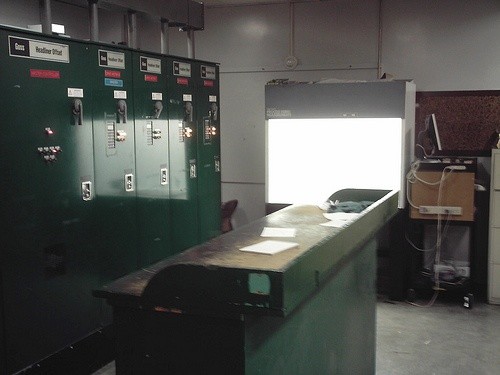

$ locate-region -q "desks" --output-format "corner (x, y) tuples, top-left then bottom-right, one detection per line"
(94, 188), (399, 375)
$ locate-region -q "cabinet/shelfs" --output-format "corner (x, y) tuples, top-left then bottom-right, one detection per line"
(1, 25), (221, 375)
